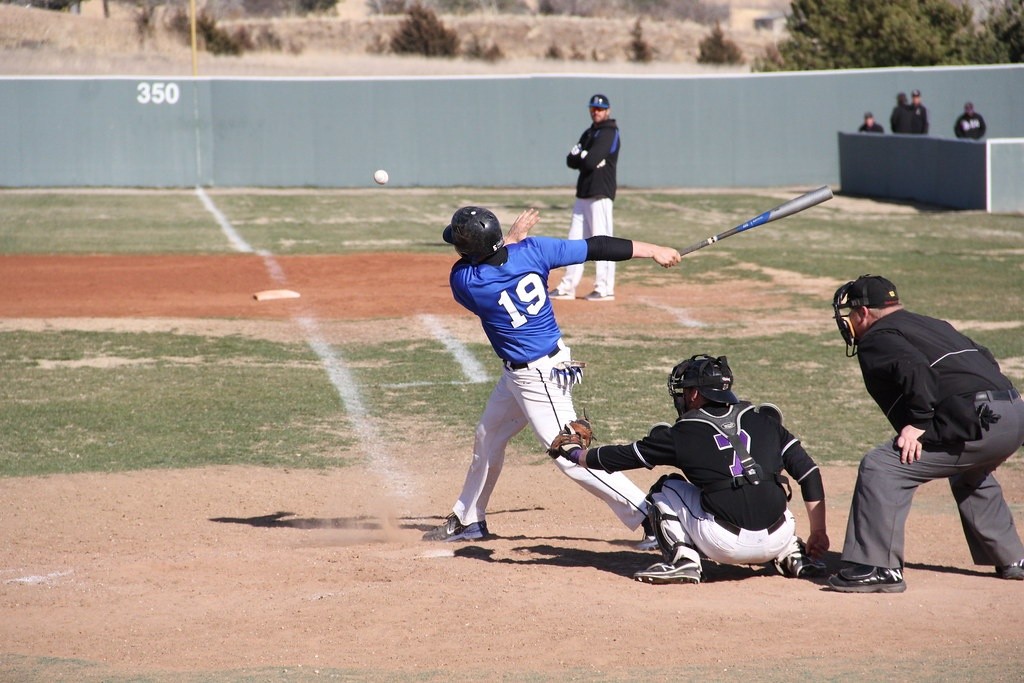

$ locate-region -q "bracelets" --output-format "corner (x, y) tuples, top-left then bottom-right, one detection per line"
(811, 529), (825, 532)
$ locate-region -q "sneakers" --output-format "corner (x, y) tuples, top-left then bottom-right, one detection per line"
(634, 559), (701, 584)
(638, 533), (661, 551)
(826, 565), (907, 594)
(996, 560), (1023, 580)
(422, 512), (490, 543)
(786, 553), (830, 578)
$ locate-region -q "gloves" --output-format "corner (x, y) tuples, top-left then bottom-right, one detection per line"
(558, 423), (582, 460)
(976, 403), (1001, 433)
(549, 359), (586, 389)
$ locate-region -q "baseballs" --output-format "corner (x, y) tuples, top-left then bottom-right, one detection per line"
(374, 170), (389, 184)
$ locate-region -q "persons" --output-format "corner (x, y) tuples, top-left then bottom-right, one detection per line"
(827, 274), (1024, 594)
(890, 89), (929, 134)
(954, 102), (986, 139)
(859, 112), (883, 133)
(547, 353), (830, 584)
(422, 205), (681, 551)
(547, 94), (620, 301)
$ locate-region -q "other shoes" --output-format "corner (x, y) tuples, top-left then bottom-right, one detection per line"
(548, 289), (576, 300)
(585, 291), (615, 301)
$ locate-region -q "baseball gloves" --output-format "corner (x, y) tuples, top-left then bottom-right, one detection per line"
(545, 406), (598, 467)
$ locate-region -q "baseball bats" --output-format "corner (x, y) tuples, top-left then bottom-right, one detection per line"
(679, 184), (834, 255)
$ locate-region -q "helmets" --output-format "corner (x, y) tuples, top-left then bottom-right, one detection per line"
(442, 206), (505, 259)
(589, 95), (610, 108)
(676, 362), (739, 403)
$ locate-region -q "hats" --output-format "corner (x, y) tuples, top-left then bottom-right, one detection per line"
(964, 102), (972, 110)
(912, 90), (920, 97)
(848, 276), (900, 308)
(864, 112), (872, 117)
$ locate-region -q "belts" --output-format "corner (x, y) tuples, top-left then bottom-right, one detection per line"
(975, 388), (1019, 400)
(503, 346), (560, 369)
(715, 515), (786, 536)
